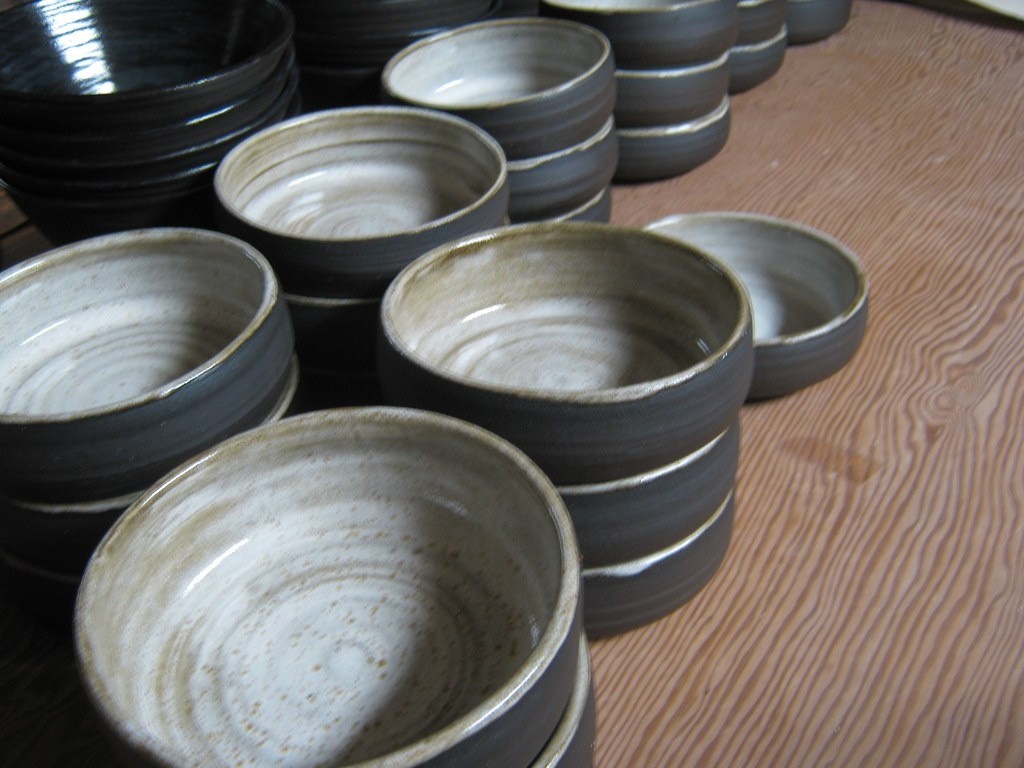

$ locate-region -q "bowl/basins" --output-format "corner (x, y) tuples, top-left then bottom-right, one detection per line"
(286, 0), (507, 110)
(382, 17), (620, 225)
(0, 0), (304, 247)
(539, 0), (852, 185)
(0, 226), (302, 624)
(205, 105), (510, 404)
(642, 212), (871, 402)
(72, 406), (598, 768)
(377, 220), (755, 645)
